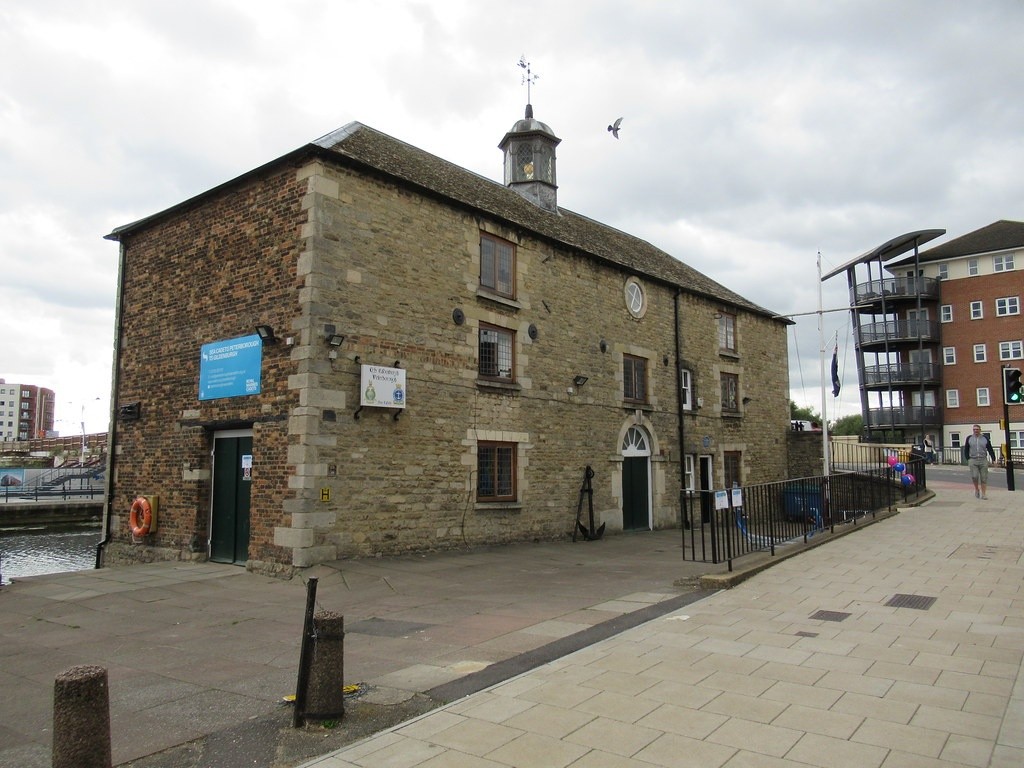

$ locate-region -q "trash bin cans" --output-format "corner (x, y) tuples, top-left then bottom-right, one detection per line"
(961, 446), (968, 466)
(912, 444), (934, 464)
(784, 483), (822, 525)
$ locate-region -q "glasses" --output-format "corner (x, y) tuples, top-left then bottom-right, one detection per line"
(973, 429), (980, 431)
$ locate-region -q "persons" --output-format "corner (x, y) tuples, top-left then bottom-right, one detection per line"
(964, 424), (997, 500)
(923, 433), (935, 465)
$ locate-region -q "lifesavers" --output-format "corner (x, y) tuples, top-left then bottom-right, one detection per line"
(129, 497), (152, 537)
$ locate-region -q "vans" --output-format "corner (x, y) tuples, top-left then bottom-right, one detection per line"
(790, 419), (823, 433)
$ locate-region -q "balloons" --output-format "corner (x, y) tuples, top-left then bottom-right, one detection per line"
(887, 455), (916, 486)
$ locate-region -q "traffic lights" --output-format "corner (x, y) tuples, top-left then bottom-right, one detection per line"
(1003, 368), (1022, 405)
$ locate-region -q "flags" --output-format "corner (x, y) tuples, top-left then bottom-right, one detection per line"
(831, 332), (841, 397)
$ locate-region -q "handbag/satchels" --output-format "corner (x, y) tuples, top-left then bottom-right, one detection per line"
(932, 448), (937, 454)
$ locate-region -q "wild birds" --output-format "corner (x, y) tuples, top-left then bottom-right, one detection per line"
(607, 117), (624, 140)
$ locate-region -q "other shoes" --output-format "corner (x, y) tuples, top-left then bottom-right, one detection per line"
(975, 489), (980, 498)
(981, 495), (988, 500)
